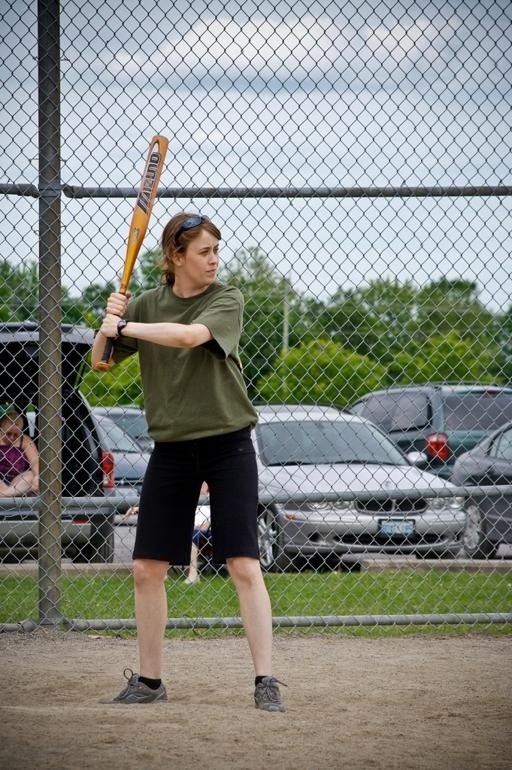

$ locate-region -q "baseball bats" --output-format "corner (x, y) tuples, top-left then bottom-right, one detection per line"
(94, 135), (168, 372)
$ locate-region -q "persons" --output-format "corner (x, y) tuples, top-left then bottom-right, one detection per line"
(1, 413), (40, 498)
(93, 215), (284, 713)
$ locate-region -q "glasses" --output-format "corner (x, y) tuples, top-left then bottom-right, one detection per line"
(175, 215), (210, 252)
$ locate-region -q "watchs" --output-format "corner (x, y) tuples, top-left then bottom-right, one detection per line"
(116, 318), (130, 339)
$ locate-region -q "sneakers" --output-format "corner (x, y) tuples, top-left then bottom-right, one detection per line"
(113, 673), (167, 703)
(255, 683), (284, 712)
